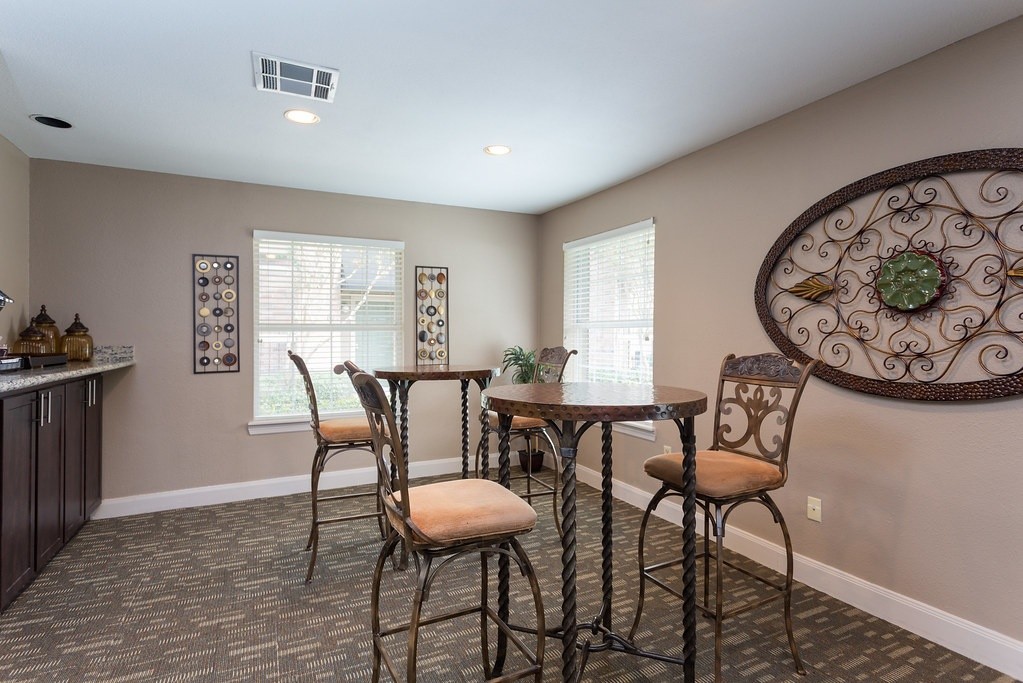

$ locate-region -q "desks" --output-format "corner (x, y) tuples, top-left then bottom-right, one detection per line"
(373, 365), (492, 571)
(481, 383), (707, 683)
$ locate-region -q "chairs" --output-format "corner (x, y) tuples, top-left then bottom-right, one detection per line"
(334, 359), (545, 683)
(476, 346), (578, 542)
(287, 350), (402, 586)
(626, 352), (825, 683)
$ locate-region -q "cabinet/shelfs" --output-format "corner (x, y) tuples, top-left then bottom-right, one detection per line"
(0, 384), (64, 609)
(65, 376), (102, 544)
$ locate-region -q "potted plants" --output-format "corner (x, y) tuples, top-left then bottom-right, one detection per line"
(501, 345), (565, 474)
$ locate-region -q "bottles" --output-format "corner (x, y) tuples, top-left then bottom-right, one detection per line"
(61, 313), (93, 361)
(34, 304), (62, 356)
(13, 317), (52, 354)
